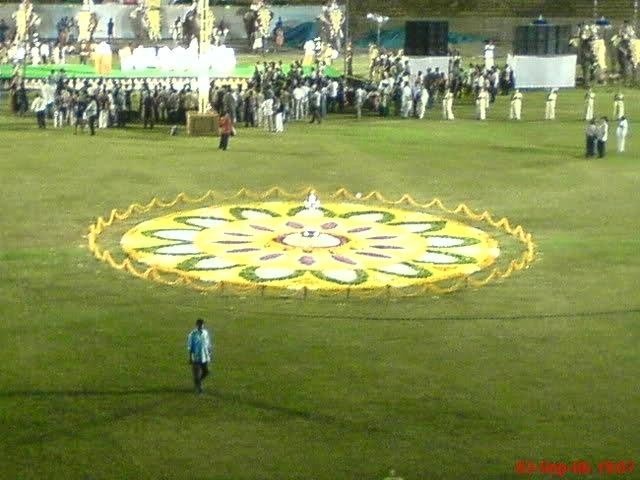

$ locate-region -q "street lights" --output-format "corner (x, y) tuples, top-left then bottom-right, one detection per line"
(366, 13), (389, 48)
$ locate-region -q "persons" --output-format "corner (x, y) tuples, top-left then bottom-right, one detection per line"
(186, 318), (213, 396)
(218, 110), (234, 149)
(0, 0), (639, 135)
(585, 118), (598, 156)
(597, 116), (609, 157)
(615, 115), (629, 154)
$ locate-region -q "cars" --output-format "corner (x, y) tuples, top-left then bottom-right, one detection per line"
(337, 76), (378, 107)
